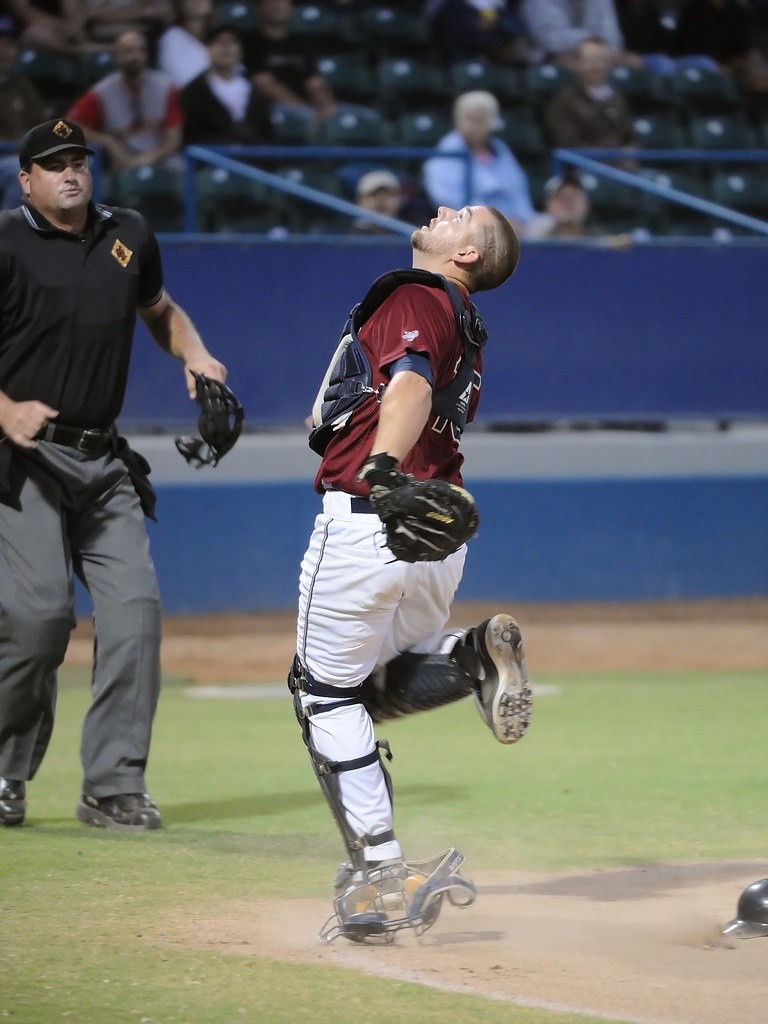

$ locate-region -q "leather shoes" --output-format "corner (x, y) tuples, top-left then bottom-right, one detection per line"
(0, 777), (26, 823)
(77, 793), (162, 832)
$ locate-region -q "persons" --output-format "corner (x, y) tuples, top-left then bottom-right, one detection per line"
(0, 116), (228, 831)
(287, 206), (533, 942)
(0, 0), (768, 238)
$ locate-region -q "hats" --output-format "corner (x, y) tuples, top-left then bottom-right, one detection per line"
(18, 119), (96, 168)
(358, 171), (400, 195)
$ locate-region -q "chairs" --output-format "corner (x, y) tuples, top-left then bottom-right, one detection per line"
(0, 0), (768, 243)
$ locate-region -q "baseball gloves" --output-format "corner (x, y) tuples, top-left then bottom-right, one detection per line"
(374, 477), (480, 566)
(173, 369), (243, 472)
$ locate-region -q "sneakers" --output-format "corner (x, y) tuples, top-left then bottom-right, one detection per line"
(448, 613), (533, 745)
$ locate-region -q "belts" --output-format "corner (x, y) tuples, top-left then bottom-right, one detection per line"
(51, 424), (113, 452)
